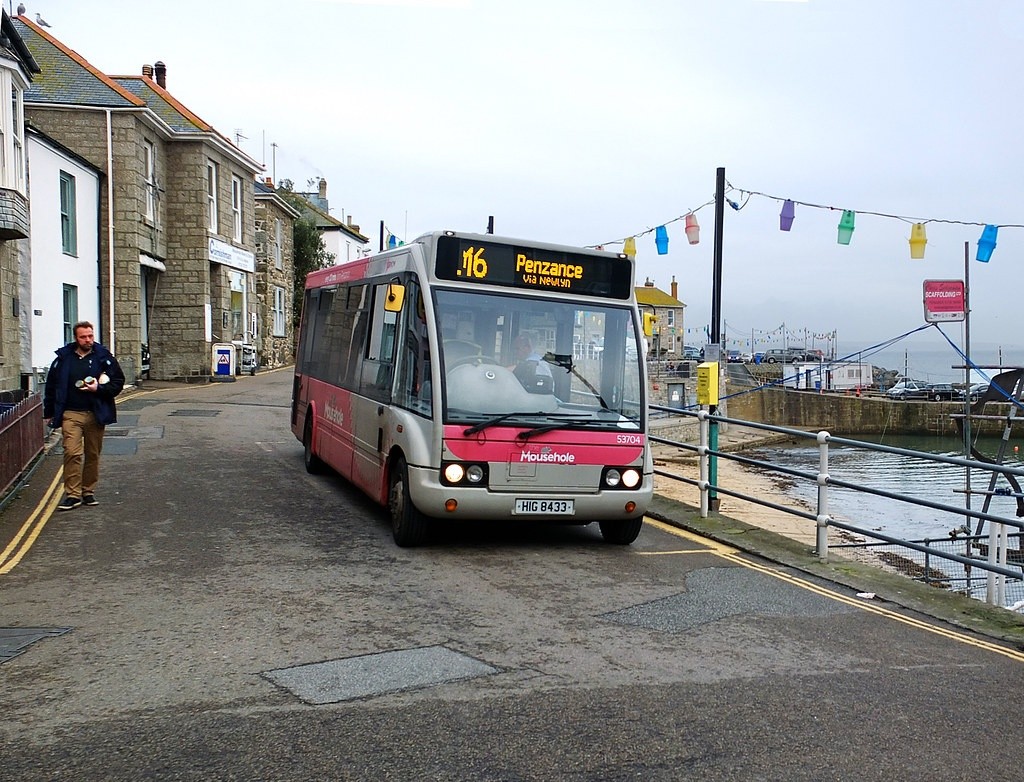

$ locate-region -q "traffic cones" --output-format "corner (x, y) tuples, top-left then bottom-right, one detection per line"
(856, 384), (860, 394)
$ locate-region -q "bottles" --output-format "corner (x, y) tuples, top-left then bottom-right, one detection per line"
(98, 370), (109, 384)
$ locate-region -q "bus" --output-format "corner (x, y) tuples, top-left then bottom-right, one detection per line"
(290, 230), (655, 544)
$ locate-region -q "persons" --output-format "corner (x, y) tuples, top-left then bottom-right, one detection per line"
(43, 322), (126, 509)
(700, 348), (705, 357)
(508, 335), (554, 394)
(666, 362), (675, 374)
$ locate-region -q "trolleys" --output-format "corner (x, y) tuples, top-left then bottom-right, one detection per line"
(231, 331), (257, 376)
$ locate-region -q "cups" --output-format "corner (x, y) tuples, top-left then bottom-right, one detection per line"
(85, 376), (94, 384)
(75, 380), (85, 388)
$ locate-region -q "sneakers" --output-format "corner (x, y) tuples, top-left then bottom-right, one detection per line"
(82, 495), (100, 506)
(57, 497), (82, 510)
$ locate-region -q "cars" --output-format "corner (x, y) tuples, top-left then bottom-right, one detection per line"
(751, 353), (765, 363)
(924, 383), (959, 401)
(958, 384), (989, 402)
(728, 350), (741, 363)
(764, 348), (823, 364)
(885, 381), (927, 400)
(740, 353), (751, 363)
(1007, 384), (1024, 402)
